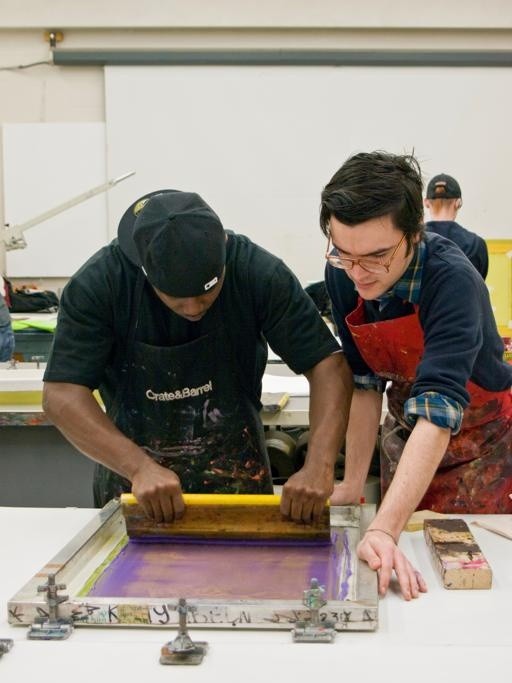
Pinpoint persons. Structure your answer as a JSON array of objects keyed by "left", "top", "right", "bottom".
[
  {"left": 40, "top": 186, "right": 357, "bottom": 525},
  {"left": 314, "top": 147, "right": 511, "bottom": 600},
  {"left": 419, "top": 171, "right": 489, "bottom": 282}
]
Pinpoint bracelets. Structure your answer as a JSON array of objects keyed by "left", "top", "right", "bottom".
[{"left": 365, "top": 526, "right": 399, "bottom": 546}]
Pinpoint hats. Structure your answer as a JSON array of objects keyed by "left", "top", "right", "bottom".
[
  {"left": 428, "top": 174, "right": 461, "bottom": 198},
  {"left": 118, "top": 189, "right": 226, "bottom": 296}
]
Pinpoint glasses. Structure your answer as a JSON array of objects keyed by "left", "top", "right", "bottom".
[{"left": 325, "top": 235, "right": 406, "bottom": 273}]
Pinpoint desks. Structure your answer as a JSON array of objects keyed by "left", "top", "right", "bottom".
[{"left": 0, "top": 310, "right": 512, "bottom": 683}]
